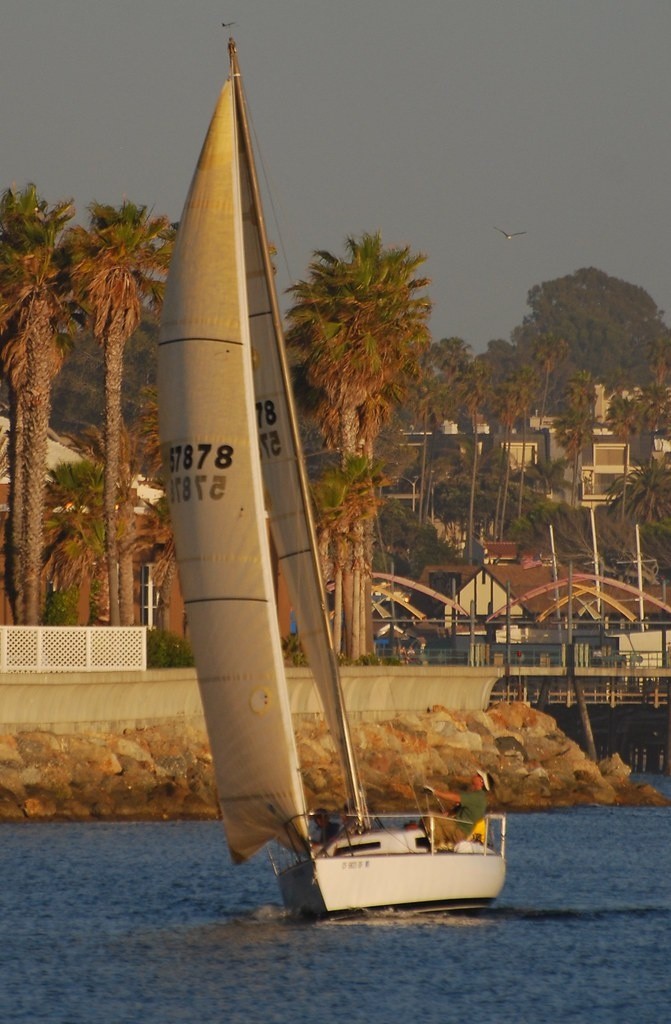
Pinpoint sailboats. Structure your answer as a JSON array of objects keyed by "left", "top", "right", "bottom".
[{"left": 157, "top": 36, "right": 509, "bottom": 917}]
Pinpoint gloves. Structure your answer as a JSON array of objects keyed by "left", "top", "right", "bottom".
[{"left": 421, "top": 786, "right": 435, "bottom": 796}]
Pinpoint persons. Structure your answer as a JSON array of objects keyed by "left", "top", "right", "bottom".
[
  {"left": 421, "top": 768, "right": 495, "bottom": 844},
  {"left": 314, "top": 808, "right": 339, "bottom": 842}
]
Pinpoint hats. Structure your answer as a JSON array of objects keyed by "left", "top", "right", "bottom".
[{"left": 478, "top": 768, "right": 494, "bottom": 793}]
